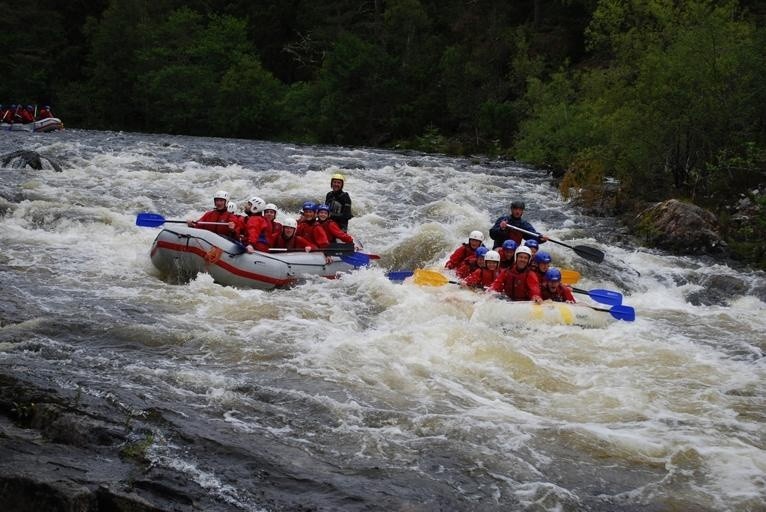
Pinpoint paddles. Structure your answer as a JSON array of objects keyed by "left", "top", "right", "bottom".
[
  {"left": 135, "top": 213, "right": 229, "bottom": 227},
  {"left": 503, "top": 218, "right": 604, "bottom": 264},
  {"left": 339, "top": 251, "right": 370, "bottom": 267},
  {"left": 382, "top": 267, "right": 450, "bottom": 279},
  {"left": 267, "top": 242, "right": 355, "bottom": 255},
  {"left": 558, "top": 271, "right": 580, "bottom": 286},
  {"left": 589, "top": 305, "right": 635, "bottom": 320},
  {"left": 364, "top": 253, "right": 381, "bottom": 260},
  {"left": 8, "top": 107, "right": 20, "bottom": 133},
  {"left": 570, "top": 286, "right": 622, "bottom": 307},
  {"left": 32, "top": 105, "right": 37, "bottom": 132},
  {"left": 414, "top": 268, "right": 486, "bottom": 292}
]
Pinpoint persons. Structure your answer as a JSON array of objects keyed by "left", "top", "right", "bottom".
[
  {"left": 186, "top": 189, "right": 354, "bottom": 266},
  {"left": 325, "top": 173, "right": 354, "bottom": 233},
  {"left": 1, "top": 105, "right": 54, "bottom": 125},
  {"left": 489, "top": 199, "right": 549, "bottom": 250},
  {"left": 444, "top": 230, "right": 576, "bottom": 304}
]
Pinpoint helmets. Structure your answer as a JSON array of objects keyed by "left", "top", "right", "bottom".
[
  {"left": 332, "top": 175, "right": 345, "bottom": 180},
  {"left": 213, "top": 190, "right": 331, "bottom": 215},
  {"left": 469, "top": 231, "right": 562, "bottom": 279},
  {"left": 510, "top": 201, "right": 524, "bottom": 207}
]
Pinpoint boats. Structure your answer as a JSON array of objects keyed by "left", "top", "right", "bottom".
[
  {"left": 404, "top": 276, "right": 611, "bottom": 331},
  {"left": 150, "top": 225, "right": 368, "bottom": 292},
  {"left": 0, "top": 118, "right": 65, "bottom": 132}
]
